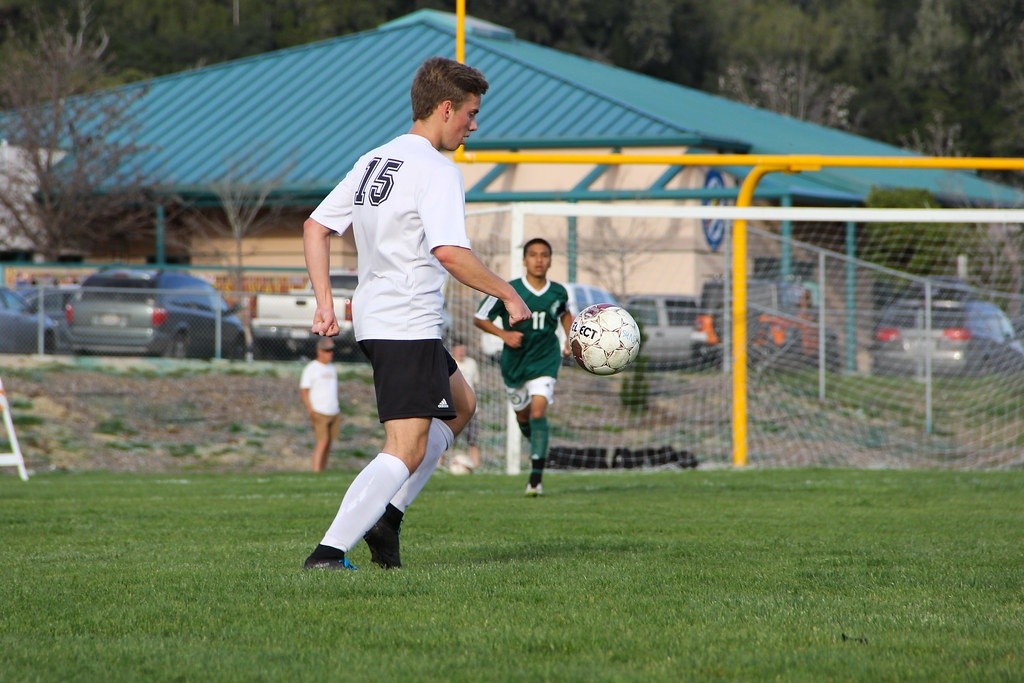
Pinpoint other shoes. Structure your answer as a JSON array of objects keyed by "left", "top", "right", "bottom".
[
  {"left": 525, "top": 473, "right": 543, "bottom": 497},
  {"left": 544, "top": 436, "right": 552, "bottom": 469}
]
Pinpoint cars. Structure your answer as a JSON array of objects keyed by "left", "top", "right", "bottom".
[{"left": 870, "top": 277, "right": 1024, "bottom": 379}]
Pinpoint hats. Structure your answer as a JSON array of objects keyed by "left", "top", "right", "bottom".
[
  {"left": 452, "top": 333, "right": 468, "bottom": 345},
  {"left": 317, "top": 336, "right": 336, "bottom": 350}
]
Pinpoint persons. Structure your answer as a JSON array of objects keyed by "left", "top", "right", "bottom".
[
  {"left": 473, "top": 238, "right": 573, "bottom": 495},
  {"left": 300, "top": 336, "right": 340, "bottom": 472},
  {"left": 441, "top": 336, "right": 481, "bottom": 474},
  {"left": 303, "top": 57, "right": 533, "bottom": 569}
]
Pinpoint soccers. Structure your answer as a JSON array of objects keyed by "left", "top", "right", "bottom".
[{"left": 571, "top": 305, "right": 642, "bottom": 373}]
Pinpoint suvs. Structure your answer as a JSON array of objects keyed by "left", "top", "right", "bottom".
[
  {"left": 480, "top": 279, "right": 838, "bottom": 373},
  {"left": 0, "top": 264, "right": 248, "bottom": 361}
]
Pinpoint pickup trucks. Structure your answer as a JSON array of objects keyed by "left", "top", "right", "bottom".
[{"left": 248, "top": 269, "right": 452, "bottom": 362}]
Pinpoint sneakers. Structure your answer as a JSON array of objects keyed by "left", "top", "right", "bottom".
[
  {"left": 304, "top": 557, "right": 360, "bottom": 574},
  {"left": 363, "top": 517, "right": 404, "bottom": 570}
]
